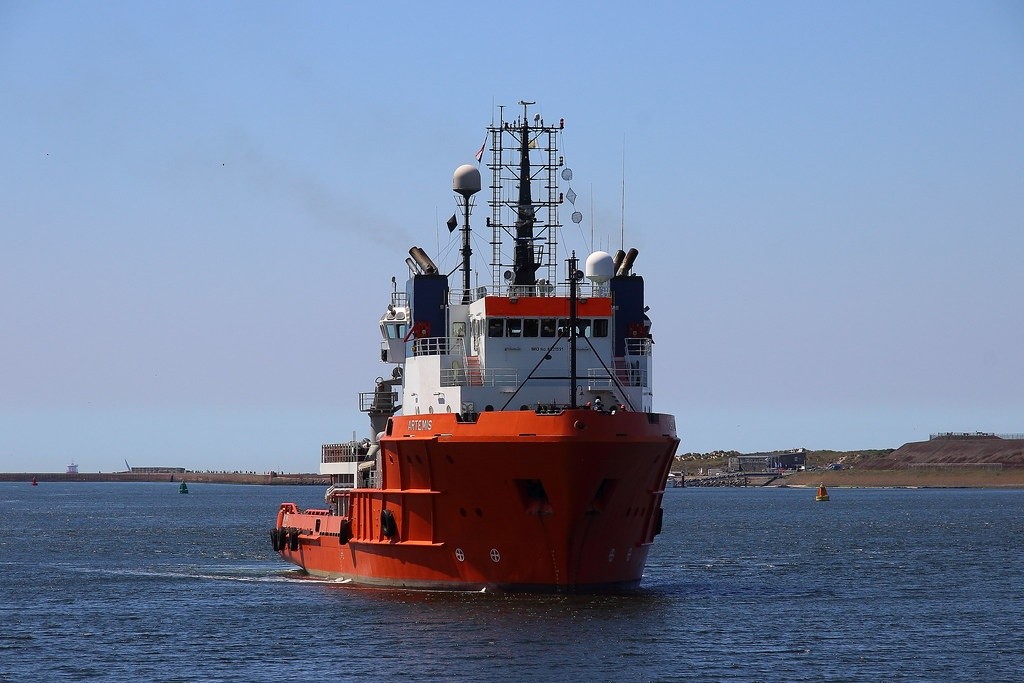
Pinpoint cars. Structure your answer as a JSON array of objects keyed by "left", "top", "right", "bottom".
[{"left": 827, "top": 464, "right": 842, "bottom": 470}]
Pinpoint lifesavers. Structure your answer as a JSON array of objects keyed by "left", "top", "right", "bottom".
[
  {"left": 269, "top": 527, "right": 279, "bottom": 553},
  {"left": 276, "top": 526, "right": 287, "bottom": 550},
  {"left": 288, "top": 527, "right": 299, "bottom": 551},
  {"left": 653, "top": 507, "right": 664, "bottom": 534},
  {"left": 380, "top": 508, "right": 396, "bottom": 537},
  {"left": 338, "top": 518, "right": 349, "bottom": 544}
]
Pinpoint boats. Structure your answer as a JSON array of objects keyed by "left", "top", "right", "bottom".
[{"left": 266, "top": 100, "right": 681, "bottom": 594}]
